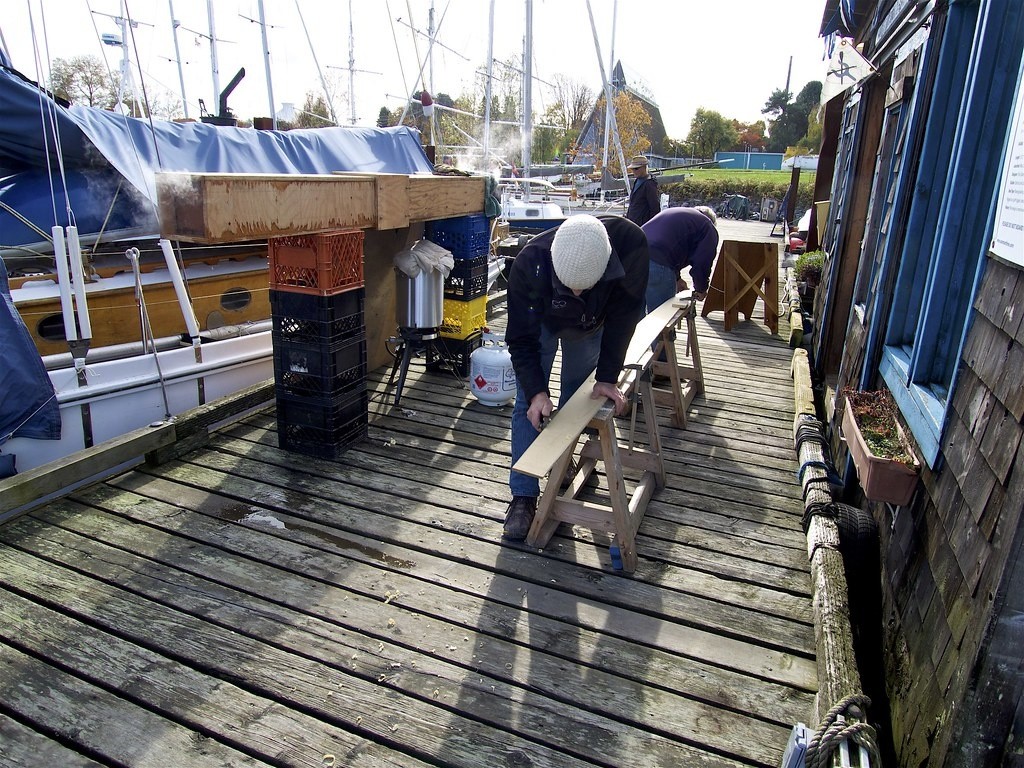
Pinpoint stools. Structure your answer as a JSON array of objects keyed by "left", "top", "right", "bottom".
[
  {"left": 612, "top": 297, "right": 705, "bottom": 430},
  {"left": 521, "top": 349, "right": 668, "bottom": 575}
]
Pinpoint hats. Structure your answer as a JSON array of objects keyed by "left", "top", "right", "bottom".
[
  {"left": 628, "top": 156, "right": 649, "bottom": 169},
  {"left": 551, "top": 214, "right": 612, "bottom": 290}
]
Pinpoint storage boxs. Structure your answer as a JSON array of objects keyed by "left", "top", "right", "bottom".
[
  {"left": 276, "top": 386, "right": 369, "bottom": 460},
  {"left": 444, "top": 255, "right": 490, "bottom": 304},
  {"left": 268, "top": 286, "right": 368, "bottom": 347},
  {"left": 266, "top": 227, "right": 367, "bottom": 297},
  {"left": 270, "top": 329, "right": 369, "bottom": 406},
  {"left": 422, "top": 213, "right": 492, "bottom": 260},
  {"left": 425, "top": 328, "right": 485, "bottom": 380},
  {"left": 438, "top": 289, "right": 488, "bottom": 340}
]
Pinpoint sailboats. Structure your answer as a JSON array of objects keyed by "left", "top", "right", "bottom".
[{"left": 0, "top": 0, "right": 668, "bottom": 525}]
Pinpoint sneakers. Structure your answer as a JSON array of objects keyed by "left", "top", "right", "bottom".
[
  {"left": 547, "top": 456, "right": 578, "bottom": 487},
  {"left": 503, "top": 496, "right": 537, "bottom": 538}
]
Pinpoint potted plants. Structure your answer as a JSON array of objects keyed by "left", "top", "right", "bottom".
[{"left": 793, "top": 248, "right": 825, "bottom": 317}]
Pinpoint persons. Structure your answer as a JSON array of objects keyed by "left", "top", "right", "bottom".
[
  {"left": 639, "top": 206, "right": 719, "bottom": 373},
  {"left": 503, "top": 216, "right": 649, "bottom": 543},
  {"left": 625, "top": 155, "right": 661, "bottom": 227}
]
